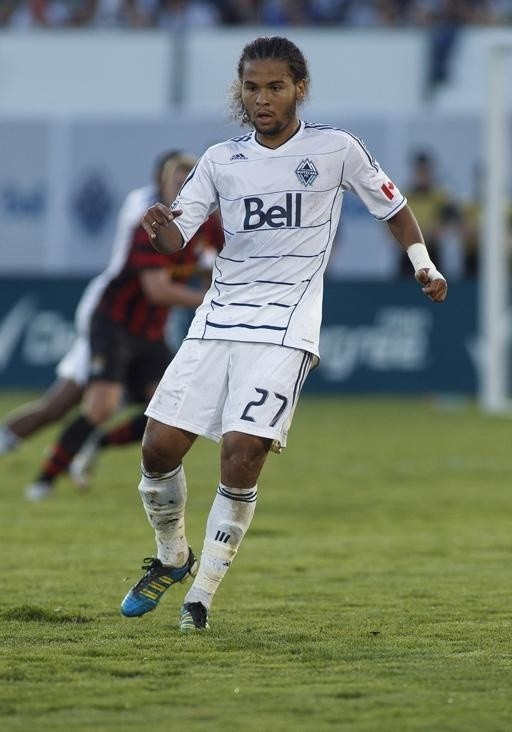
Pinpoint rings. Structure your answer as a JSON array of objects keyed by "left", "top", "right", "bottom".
[{"left": 151, "top": 221, "right": 157, "bottom": 226}]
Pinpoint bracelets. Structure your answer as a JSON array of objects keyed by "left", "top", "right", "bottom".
[{"left": 405, "top": 243, "right": 437, "bottom": 271}]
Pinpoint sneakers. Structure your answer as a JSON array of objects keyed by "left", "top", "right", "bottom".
[
  {"left": 179, "top": 601, "right": 210, "bottom": 636},
  {"left": 24, "top": 441, "right": 99, "bottom": 503},
  {"left": 120, "top": 545, "right": 198, "bottom": 618}
]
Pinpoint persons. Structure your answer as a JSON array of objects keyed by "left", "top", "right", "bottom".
[
  {"left": 1, "top": 1, "right": 511, "bottom": 30},
  {"left": 397, "top": 152, "right": 452, "bottom": 284},
  {"left": 118, "top": 35, "right": 450, "bottom": 633},
  {"left": 459, "top": 157, "right": 478, "bottom": 275},
  {"left": 0, "top": 150, "right": 184, "bottom": 449},
  {"left": 21, "top": 159, "right": 225, "bottom": 503}
]
